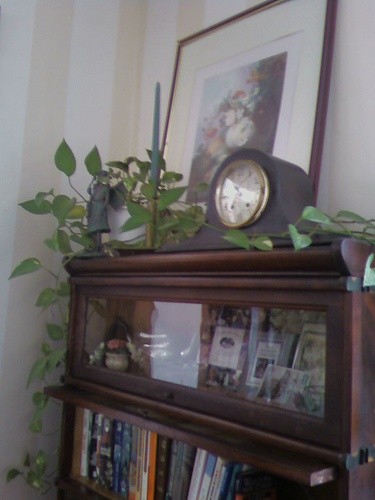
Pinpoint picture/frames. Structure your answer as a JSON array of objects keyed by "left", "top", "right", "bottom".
[{"left": 156, "top": 0, "right": 336, "bottom": 249}]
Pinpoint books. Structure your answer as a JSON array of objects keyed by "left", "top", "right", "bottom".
[{"left": 69, "top": 406, "right": 248, "bottom": 500}]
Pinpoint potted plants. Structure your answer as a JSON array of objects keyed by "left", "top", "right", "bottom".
[{"left": 5, "top": 137, "right": 374, "bottom": 492}]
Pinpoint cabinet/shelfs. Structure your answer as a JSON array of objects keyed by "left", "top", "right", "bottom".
[{"left": 42, "top": 237, "right": 374, "bottom": 500}]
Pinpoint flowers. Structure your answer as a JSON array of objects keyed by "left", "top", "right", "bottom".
[{"left": 107, "top": 338, "right": 127, "bottom": 352}]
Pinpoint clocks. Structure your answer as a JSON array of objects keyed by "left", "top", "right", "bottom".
[{"left": 192, "top": 149, "right": 308, "bottom": 244}]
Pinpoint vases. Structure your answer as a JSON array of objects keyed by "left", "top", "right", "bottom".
[{"left": 106, "top": 354, "right": 129, "bottom": 372}]
